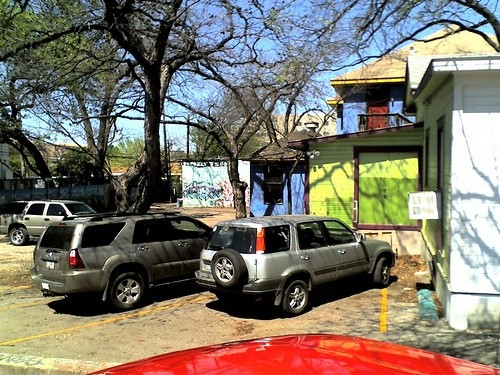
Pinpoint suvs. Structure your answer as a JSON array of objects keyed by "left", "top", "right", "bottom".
[
  {"left": 28, "top": 209, "right": 215, "bottom": 311},
  {"left": 196, "top": 211, "right": 396, "bottom": 312},
  {"left": 1, "top": 200, "right": 95, "bottom": 244}
]
